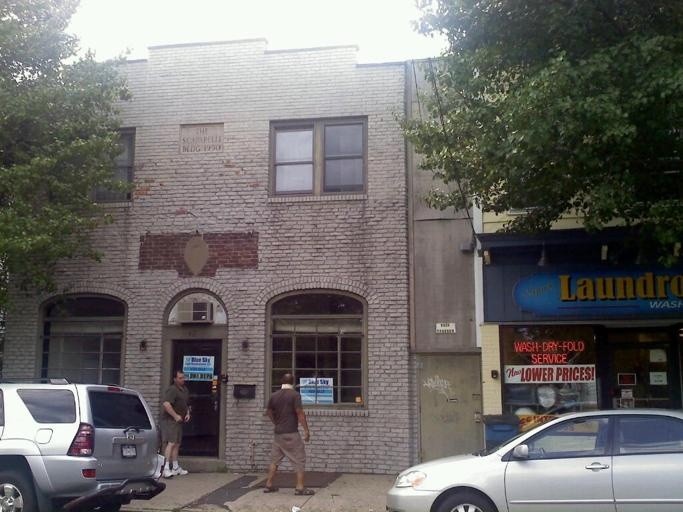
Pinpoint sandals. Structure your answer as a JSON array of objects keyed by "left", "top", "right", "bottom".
[
  {"left": 263, "top": 486, "right": 279, "bottom": 493},
  {"left": 295, "top": 487, "right": 314, "bottom": 496}
]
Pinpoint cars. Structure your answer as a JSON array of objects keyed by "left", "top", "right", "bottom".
[
  {"left": 386, "top": 409, "right": 683, "bottom": 512},
  {"left": 0, "top": 383, "right": 166, "bottom": 512}
]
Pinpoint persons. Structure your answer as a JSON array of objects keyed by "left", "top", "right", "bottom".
[
  {"left": 263, "top": 373, "right": 315, "bottom": 494},
  {"left": 160, "top": 371, "right": 192, "bottom": 477}
]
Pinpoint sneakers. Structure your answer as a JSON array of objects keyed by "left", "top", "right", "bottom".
[
  {"left": 162, "top": 470, "right": 172, "bottom": 478},
  {"left": 172, "top": 466, "right": 188, "bottom": 475}
]
